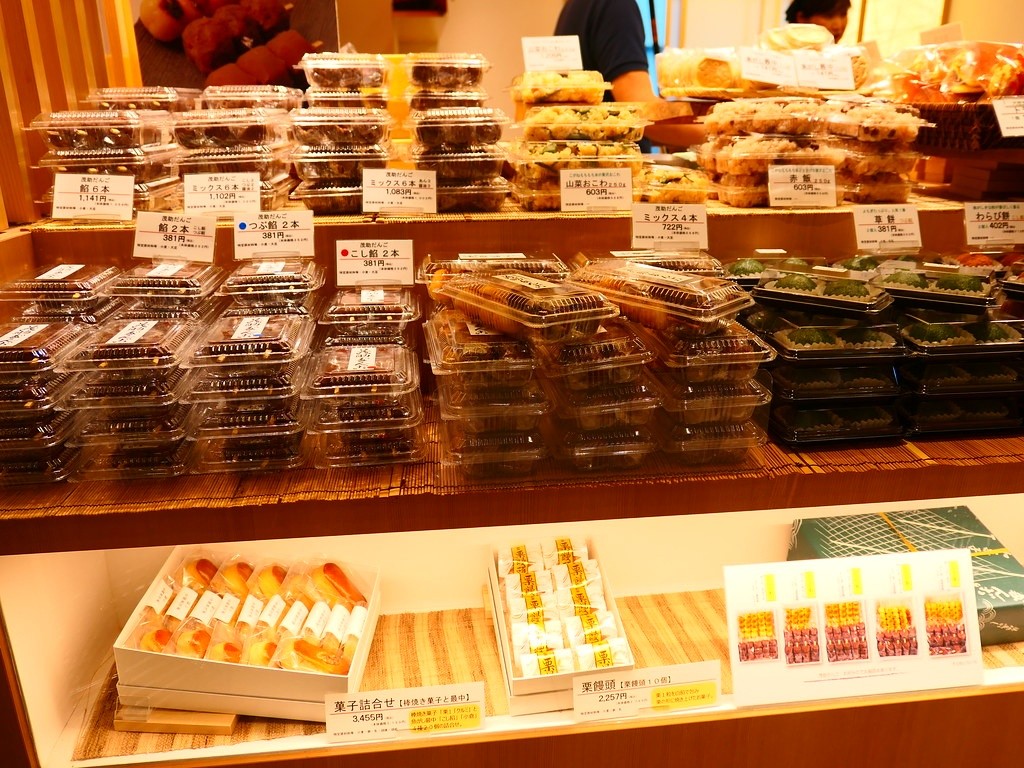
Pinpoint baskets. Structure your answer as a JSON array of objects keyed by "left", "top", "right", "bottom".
[{"left": 911, "top": 101, "right": 1024, "bottom": 152}]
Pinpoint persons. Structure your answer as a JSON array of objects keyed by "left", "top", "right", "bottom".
[
  {"left": 556, "top": 0, "right": 706, "bottom": 152},
  {"left": 786, "top": 0, "right": 851, "bottom": 44}
]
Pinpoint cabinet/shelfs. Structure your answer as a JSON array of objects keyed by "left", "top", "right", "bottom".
[{"left": 0, "top": 189, "right": 1024, "bottom": 768}]
